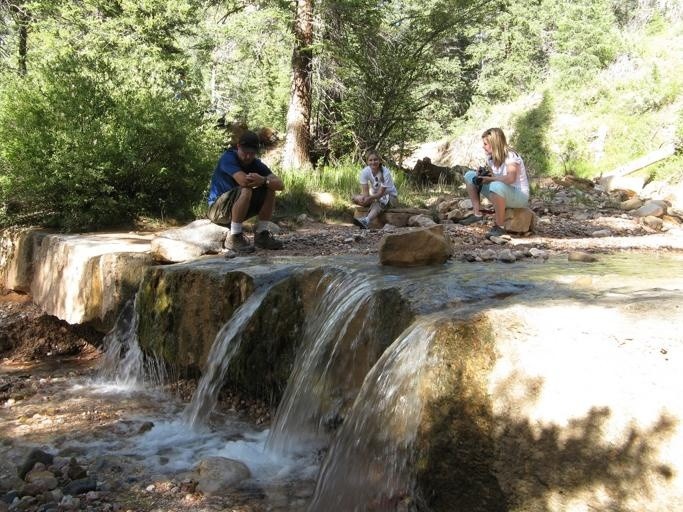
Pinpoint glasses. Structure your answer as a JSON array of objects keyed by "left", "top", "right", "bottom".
[{"left": 374, "top": 177, "right": 378, "bottom": 188}]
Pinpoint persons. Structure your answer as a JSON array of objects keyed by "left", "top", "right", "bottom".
[
  {"left": 204, "top": 130, "right": 286, "bottom": 252},
  {"left": 345, "top": 149, "right": 398, "bottom": 228},
  {"left": 453, "top": 127, "right": 528, "bottom": 241}
]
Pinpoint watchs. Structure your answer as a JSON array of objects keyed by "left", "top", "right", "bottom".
[{"left": 264, "top": 177, "right": 268, "bottom": 186}]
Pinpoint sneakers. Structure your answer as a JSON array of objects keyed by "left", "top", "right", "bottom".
[
  {"left": 254, "top": 230, "right": 282, "bottom": 249},
  {"left": 352, "top": 217, "right": 369, "bottom": 230},
  {"left": 224, "top": 232, "right": 255, "bottom": 253},
  {"left": 485, "top": 225, "right": 506, "bottom": 240},
  {"left": 459, "top": 214, "right": 483, "bottom": 226}
]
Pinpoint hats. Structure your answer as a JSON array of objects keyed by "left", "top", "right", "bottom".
[{"left": 239, "top": 131, "right": 259, "bottom": 153}]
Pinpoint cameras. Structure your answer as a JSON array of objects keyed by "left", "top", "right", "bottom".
[{"left": 473, "top": 166, "right": 491, "bottom": 186}]
